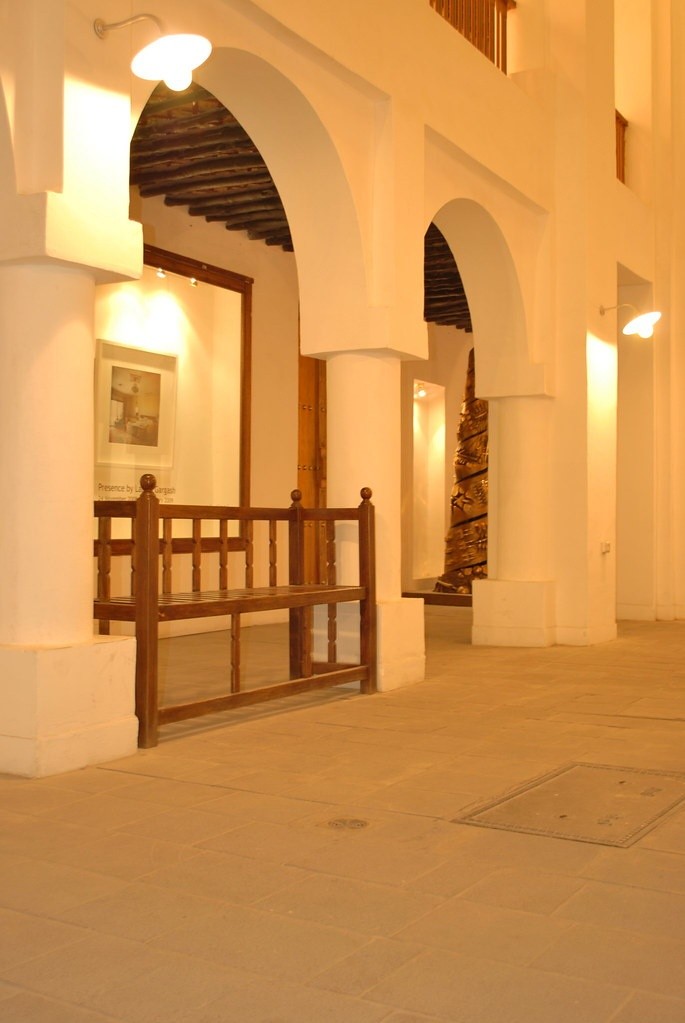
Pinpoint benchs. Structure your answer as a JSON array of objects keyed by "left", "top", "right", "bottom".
[{"left": 94, "top": 477, "right": 380, "bottom": 747}]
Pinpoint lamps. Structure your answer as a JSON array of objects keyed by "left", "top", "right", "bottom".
[
  {"left": 600, "top": 303, "right": 662, "bottom": 338},
  {"left": 94, "top": 14, "right": 213, "bottom": 91}
]
